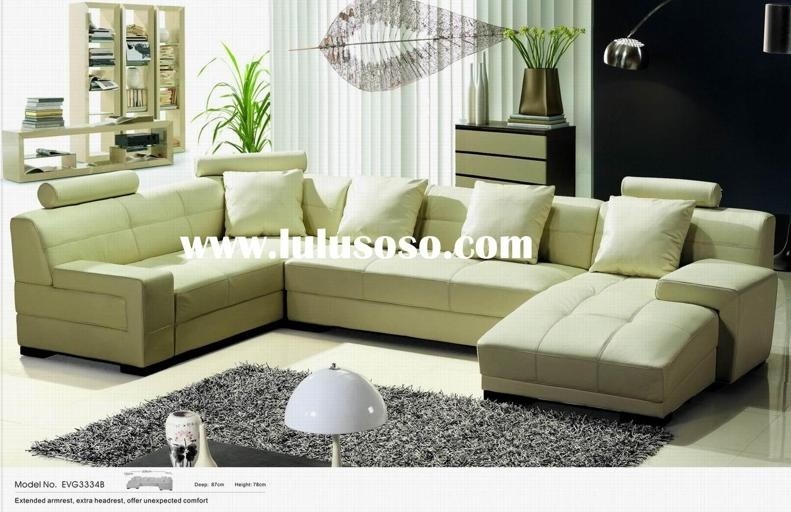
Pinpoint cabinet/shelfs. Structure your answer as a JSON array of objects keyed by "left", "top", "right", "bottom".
[
  {"left": 2, "top": 0, "right": 185, "bottom": 184},
  {"left": 455, "top": 120, "right": 576, "bottom": 197}
]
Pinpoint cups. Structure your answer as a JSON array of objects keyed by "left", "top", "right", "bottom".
[
  {"left": 164, "top": 411, "right": 201, "bottom": 467},
  {"left": 110, "top": 146, "right": 127, "bottom": 163}
]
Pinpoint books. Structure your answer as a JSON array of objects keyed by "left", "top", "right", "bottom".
[
  {"left": 89, "top": 25, "right": 115, "bottom": 67},
  {"left": 160, "top": 37, "right": 178, "bottom": 106},
  {"left": 127, "top": 87, "right": 147, "bottom": 108},
  {"left": 126, "top": 36, "right": 151, "bottom": 65},
  {"left": 21, "top": 97, "right": 65, "bottom": 131},
  {"left": 109, "top": 116, "right": 153, "bottom": 123},
  {"left": 507, "top": 114, "right": 570, "bottom": 130}
]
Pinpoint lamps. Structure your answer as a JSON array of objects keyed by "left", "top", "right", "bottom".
[
  {"left": 760, "top": 3, "right": 790, "bottom": 53},
  {"left": 603, "top": 1, "right": 669, "bottom": 71},
  {"left": 285, "top": 363, "right": 387, "bottom": 467}
]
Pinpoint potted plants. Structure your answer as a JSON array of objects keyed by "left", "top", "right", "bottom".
[{"left": 503, "top": 23, "right": 586, "bottom": 118}]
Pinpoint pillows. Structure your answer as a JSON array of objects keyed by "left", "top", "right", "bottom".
[
  {"left": 585, "top": 195, "right": 695, "bottom": 278},
  {"left": 336, "top": 175, "right": 429, "bottom": 248},
  {"left": 221, "top": 168, "right": 308, "bottom": 239},
  {"left": 454, "top": 177, "right": 556, "bottom": 265}
]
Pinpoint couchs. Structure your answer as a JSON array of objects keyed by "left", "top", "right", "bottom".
[{"left": 10, "top": 149, "right": 777, "bottom": 421}]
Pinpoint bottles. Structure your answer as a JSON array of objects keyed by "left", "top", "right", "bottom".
[
  {"left": 126, "top": 67, "right": 141, "bottom": 88},
  {"left": 465, "top": 50, "right": 490, "bottom": 126},
  {"left": 192, "top": 424, "right": 218, "bottom": 467}
]
